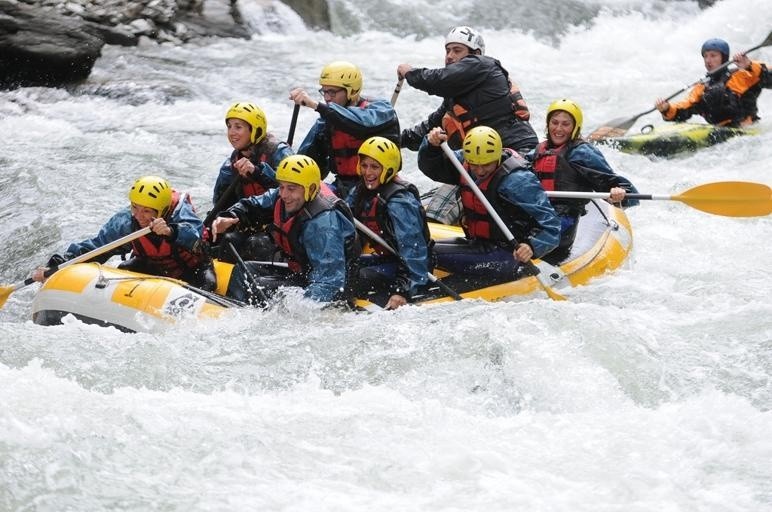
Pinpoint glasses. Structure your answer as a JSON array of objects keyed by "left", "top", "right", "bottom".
[{"left": 318, "top": 87, "right": 343, "bottom": 96}]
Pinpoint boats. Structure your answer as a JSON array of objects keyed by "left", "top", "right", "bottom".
[
  {"left": 594, "top": 110, "right": 772, "bottom": 161},
  {"left": 31, "top": 193, "right": 636, "bottom": 336}
]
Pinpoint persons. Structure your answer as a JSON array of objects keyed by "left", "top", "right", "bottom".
[
  {"left": 418, "top": 125, "right": 562, "bottom": 285},
  {"left": 204, "top": 103, "right": 297, "bottom": 266},
  {"left": 32, "top": 176, "right": 217, "bottom": 294},
  {"left": 210, "top": 154, "right": 362, "bottom": 312},
  {"left": 343, "top": 136, "right": 438, "bottom": 310},
  {"left": 655, "top": 39, "right": 772, "bottom": 128},
  {"left": 524, "top": 98, "right": 640, "bottom": 266},
  {"left": 289, "top": 62, "right": 402, "bottom": 200},
  {"left": 399, "top": 26, "right": 539, "bottom": 225}
]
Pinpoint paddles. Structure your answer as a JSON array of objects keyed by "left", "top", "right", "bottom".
[
  {"left": 1, "top": 226, "right": 153, "bottom": 308},
  {"left": 441, "top": 132, "right": 573, "bottom": 300},
  {"left": 586, "top": 30, "right": 772, "bottom": 141},
  {"left": 545, "top": 181, "right": 771, "bottom": 217}
]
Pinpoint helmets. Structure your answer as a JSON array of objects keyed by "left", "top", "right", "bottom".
[
  {"left": 546, "top": 98, "right": 583, "bottom": 141},
  {"left": 701, "top": 39, "right": 729, "bottom": 64},
  {"left": 445, "top": 26, "right": 485, "bottom": 55},
  {"left": 356, "top": 136, "right": 401, "bottom": 185},
  {"left": 463, "top": 125, "right": 502, "bottom": 167},
  {"left": 226, "top": 102, "right": 266, "bottom": 145},
  {"left": 129, "top": 176, "right": 173, "bottom": 218},
  {"left": 276, "top": 154, "right": 321, "bottom": 202},
  {"left": 319, "top": 62, "right": 362, "bottom": 101}
]
